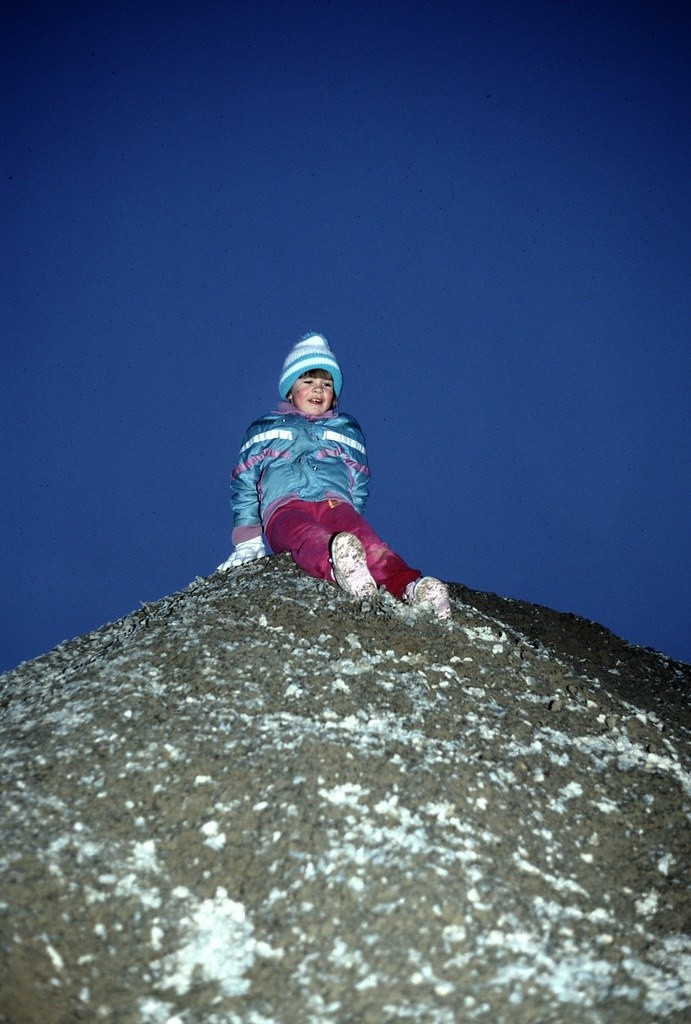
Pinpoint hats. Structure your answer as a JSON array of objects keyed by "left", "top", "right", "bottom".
[{"left": 279, "top": 331, "right": 342, "bottom": 401}]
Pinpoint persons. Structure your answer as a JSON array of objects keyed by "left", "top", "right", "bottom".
[{"left": 217, "top": 332, "right": 451, "bottom": 620}]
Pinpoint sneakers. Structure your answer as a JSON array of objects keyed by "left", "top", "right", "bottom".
[
  {"left": 404, "top": 576, "right": 452, "bottom": 621},
  {"left": 329, "top": 531, "right": 377, "bottom": 598},
  {"left": 217, "top": 535, "right": 266, "bottom": 571}
]
